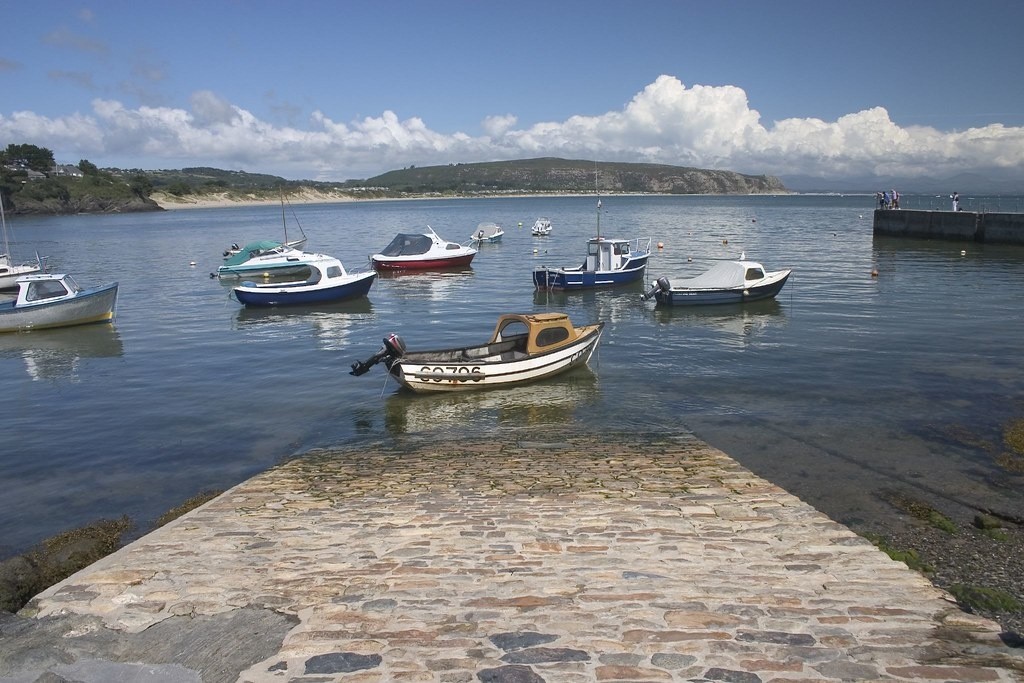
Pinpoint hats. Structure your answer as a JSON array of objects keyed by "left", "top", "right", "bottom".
[{"left": 953, "top": 192, "right": 957, "bottom": 194}]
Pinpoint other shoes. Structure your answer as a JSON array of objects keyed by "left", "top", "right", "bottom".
[
  {"left": 891, "top": 208, "right": 895, "bottom": 210},
  {"left": 896, "top": 208, "right": 900, "bottom": 210}
]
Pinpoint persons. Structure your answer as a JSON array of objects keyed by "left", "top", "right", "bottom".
[
  {"left": 877, "top": 189, "right": 901, "bottom": 210},
  {"left": 952, "top": 191, "right": 959, "bottom": 212}
]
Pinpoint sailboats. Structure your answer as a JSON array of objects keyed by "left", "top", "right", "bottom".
[{"left": 0, "top": 192, "right": 41, "bottom": 293}]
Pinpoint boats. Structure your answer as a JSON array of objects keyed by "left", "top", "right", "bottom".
[
  {"left": 209, "top": 183, "right": 378, "bottom": 306},
  {"left": 348, "top": 312, "right": 606, "bottom": 394},
  {"left": 371, "top": 224, "right": 481, "bottom": 271},
  {"left": 640, "top": 249, "right": 792, "bottom": 307},
  {"left": 531, "top": 216, "right": 552, "bottom": 236},
  {"left": 533, "top": 196, "right": 652, "bottom": 291},
  {"left": 470, "top": 222, "right": 504, "bottom": 244},
  {"left": 0, "top": 275, "right": 118, "bottom": 334}
]
